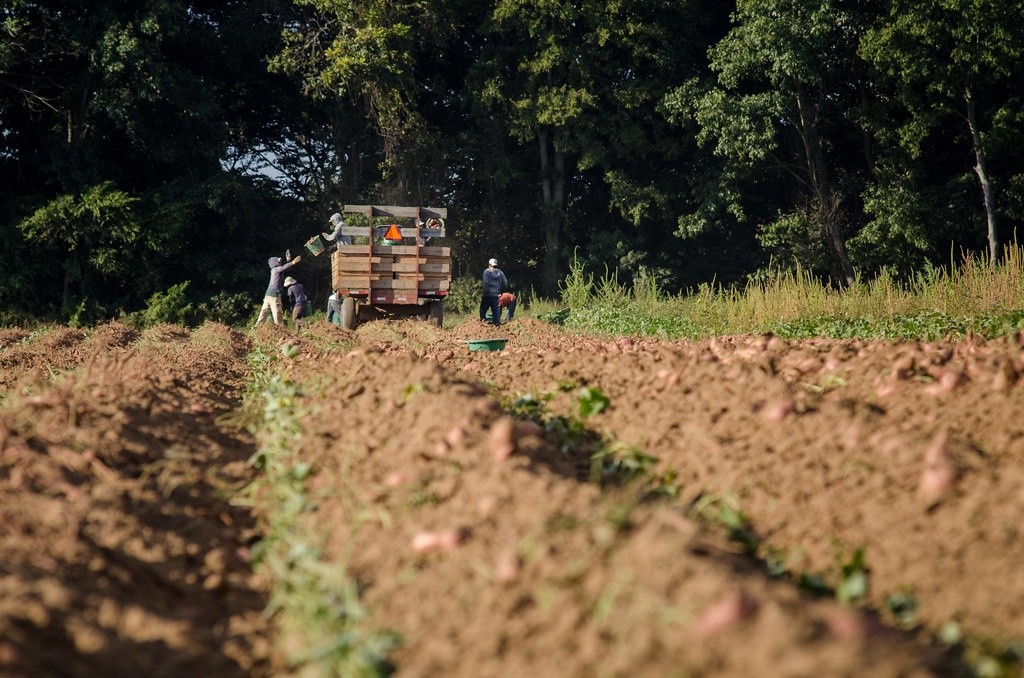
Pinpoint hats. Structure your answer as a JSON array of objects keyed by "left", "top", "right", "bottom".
[
  {"left": 489, "top": 259, "right": 498, "bottom": 266},
  {"left": 284, "top": 276, "right": 298, "bottom": 286},
  {"left": 328, "top": 212, "right": 342, "bottom": 222},
  {"left": 268, "top": 257, "right": 280, "bottom": 268}
]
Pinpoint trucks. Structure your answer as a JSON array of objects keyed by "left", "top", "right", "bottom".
[{"left": 330, "top": 205, "right": 451, "bottom": 329}]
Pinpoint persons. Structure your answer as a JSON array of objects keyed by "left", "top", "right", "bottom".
[
  {"left": 496, "top": 292, "right": 517, "bottom": 320},
  {"left": 479, "top": 258, "right": 508, "bottom": 325},
  {"left": 416, "top": 220, "right": 432, "bottom": 247},
  {"left": 284, "top": 276, "right": 311, "bottom": 319},
  {"left": 253, "top": 256, "right": 302, "bottom": 327},
  {"left": 322, "top": 213, "right": 353, "bottom": 250}
]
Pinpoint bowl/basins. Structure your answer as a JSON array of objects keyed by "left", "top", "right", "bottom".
[{"left": 466, "top": 339, "right": 507, "bottom": 352}]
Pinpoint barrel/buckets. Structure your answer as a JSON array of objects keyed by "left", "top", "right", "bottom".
[
  {"left": 303, "top": 303, "right": 312, "bottom": 317},
  {"left": 332, "top": 312, "right": 341, "bottom": 326},
  {"left": 303, "top": 235, "right": 325, "bottom": 256},
  {"left": 426, "top": 218, "right": 443, "bottom": 230}
]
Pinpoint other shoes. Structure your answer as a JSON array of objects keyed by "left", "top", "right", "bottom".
[{"left": 493, "top": 322, "right": 501, "bottom": 326}]
